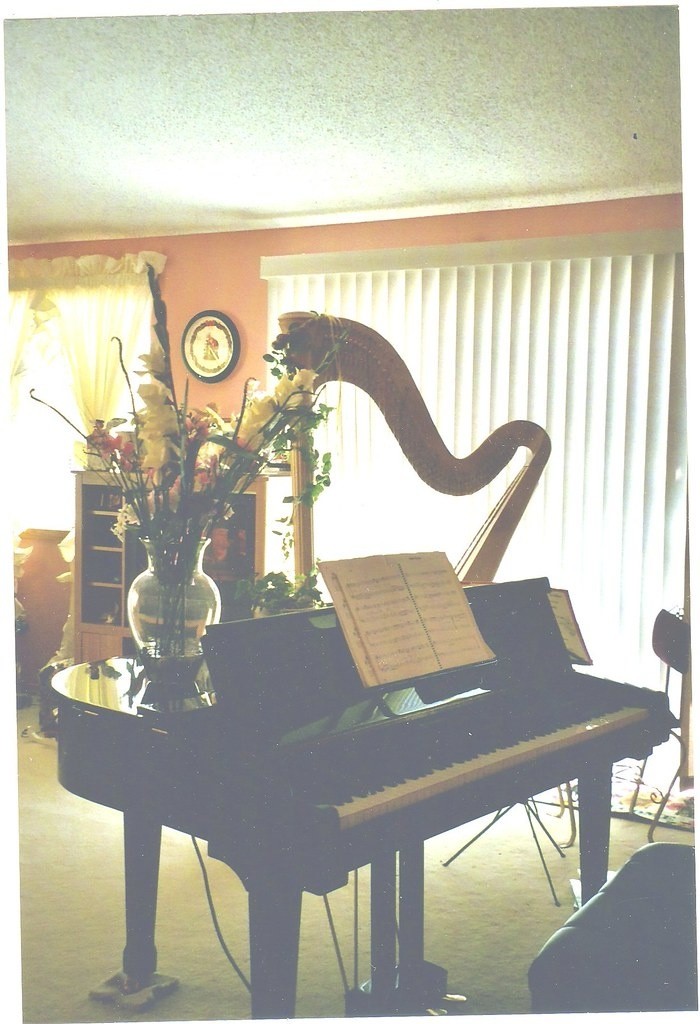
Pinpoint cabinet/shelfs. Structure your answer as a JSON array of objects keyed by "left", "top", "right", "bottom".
[{"left": 72, "top": 472, "right": 269, "bottom": 664}]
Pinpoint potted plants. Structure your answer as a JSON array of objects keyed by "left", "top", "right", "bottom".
[{"left": 234, "top": 558, "right": 327, "bottom": 618}]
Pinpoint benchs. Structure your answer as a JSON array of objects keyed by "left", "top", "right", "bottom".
[{"left": 527, "top": 842, "right": 698, "bottom": 1011}]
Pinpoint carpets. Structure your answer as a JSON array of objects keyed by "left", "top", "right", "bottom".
[{"left": 529, "top": 780, "right": 695, "bottom": 833}]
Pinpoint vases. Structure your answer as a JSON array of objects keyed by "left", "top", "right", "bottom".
[
  {"left": 181, "top": 310, "right": 240, "bottom": 384},
  {"left": 127, "top": 535, "right": 223, "bottom": 681}
]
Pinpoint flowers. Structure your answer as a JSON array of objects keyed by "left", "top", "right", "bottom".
[{"left": 29, "top": 262, "right": 337, "bottom": 660}]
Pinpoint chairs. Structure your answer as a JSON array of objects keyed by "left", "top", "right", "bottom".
[{"left": 555, "top": 610, "right": 691, "bottom": 848}]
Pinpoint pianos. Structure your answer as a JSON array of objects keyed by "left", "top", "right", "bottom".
[{"left": 45, "top": 576, "right": 680, "bottom": 1019}]
